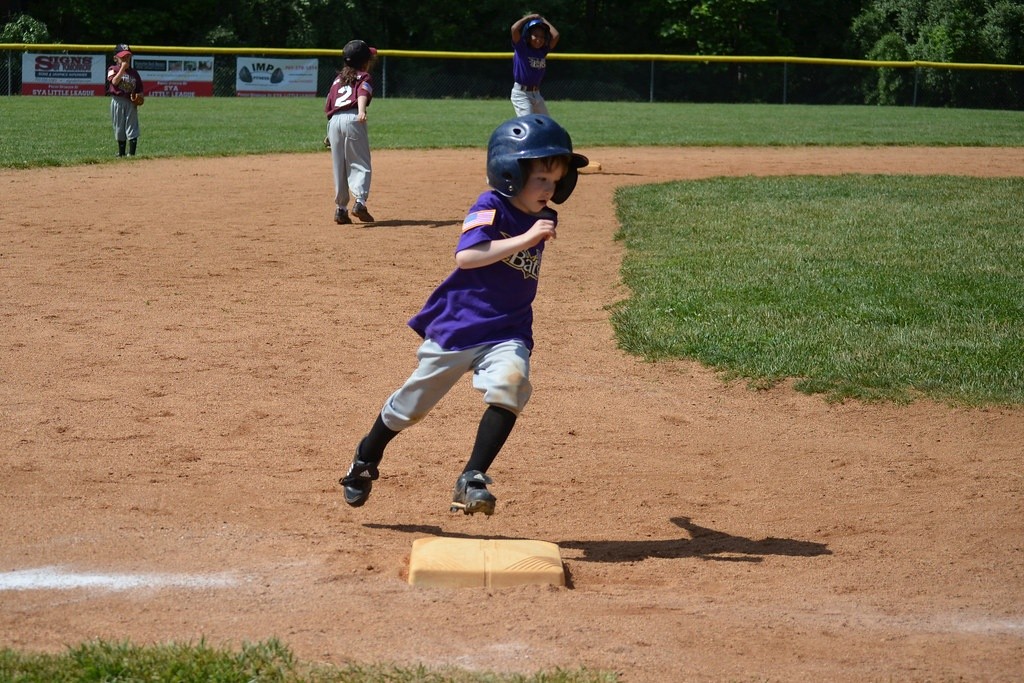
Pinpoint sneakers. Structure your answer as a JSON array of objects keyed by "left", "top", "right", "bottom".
[
  {"left": 449, "top": 470, "right": 497, "bottom": 520},
  {"left": 338, "top": 438, "right": 379, "bottom": 508}
]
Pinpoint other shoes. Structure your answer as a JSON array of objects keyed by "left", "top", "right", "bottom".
[
  {"left": 334, "top": 209, "right": 352, "bottom": 224},
  {"left": 351, "top": 202, "right": 374, "bottom": 223}
]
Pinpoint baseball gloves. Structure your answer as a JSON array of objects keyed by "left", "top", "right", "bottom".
[{"left": 130, "top": 93, "right": 144, "bottom": 105}]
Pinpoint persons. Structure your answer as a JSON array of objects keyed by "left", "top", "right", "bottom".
[
  {"left": 107, "top": 45, "right": 144, "bottom": 156},
  {"left": 339, "top": 113, "right": 589, "bottom": 516},
  {"left": 511, "top": 14, "right": 559, "bottom": 117},
  {"left": 323, "top": 40, "right": 377, "bottom": 224}
]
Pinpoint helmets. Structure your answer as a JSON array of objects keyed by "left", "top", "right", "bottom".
[
  {"left": 522, "top": 19, "right": 550, "bottom": 42},
  {"left": 486, "top": 114, "right": 589, "bottom": 204}
]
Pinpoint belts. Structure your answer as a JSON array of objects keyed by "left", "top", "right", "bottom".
[{"left": 521, "top": 85, "right": 538, "bottom": 91}]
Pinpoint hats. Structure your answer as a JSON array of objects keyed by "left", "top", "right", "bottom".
[
  {"left": 343, "top": 40, "right": 377, "bottom": 65},
  {"left": 114, "top": 44, "right": 132, "bottom": 58}
]
